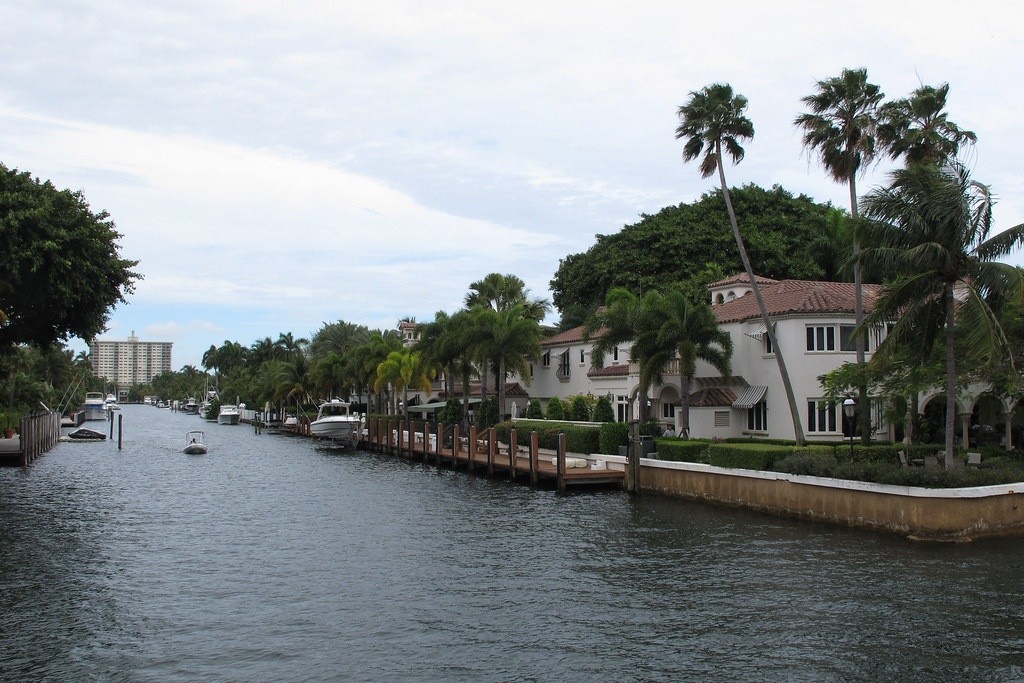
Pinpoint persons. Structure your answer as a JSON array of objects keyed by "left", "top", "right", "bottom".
[{"left": 663, "top": 424, "right": 674, "bottom": 437}]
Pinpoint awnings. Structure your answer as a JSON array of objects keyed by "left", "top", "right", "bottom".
[
  {"left": 732, "top": 385, "right": 768, "bottom": 410},
  {"left": 407, "top": 398, "right": 491, "bottom": 412},
  {"left": 748, "top": 321, "right": 778, "bottom": 338}
]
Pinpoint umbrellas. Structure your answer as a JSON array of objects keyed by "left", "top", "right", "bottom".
[{"left": 510, "top": 402, "right": 517, "bottom": 422}]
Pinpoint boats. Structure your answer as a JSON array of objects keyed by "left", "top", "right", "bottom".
[
  {"left": 217, "top": 403, "right": 241, "bottom": 426},
  {"left": 68, "top": 426, "right": 107, "bottom": 440},
  {"left": 156, "top": 376, "right": 219, "bottom": 418},
  {"left": 104, "top": 392, "right": 122, "bottom": 411},
  {"left": 183, "top": 430, "right": 209, "bottom": 454},
  {"left": 310, "top": 396, "right": 362, "bottom": 444},
  {"left": 82, "top": 391, "right": 109, "bottom": 421},
  {"left": 284, "top": 416, "right": 298, "bottom": 429}
]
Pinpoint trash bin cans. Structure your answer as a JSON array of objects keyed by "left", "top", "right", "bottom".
[{"left": 637, "top": 434, "right": 657, "bottom": 459}]
